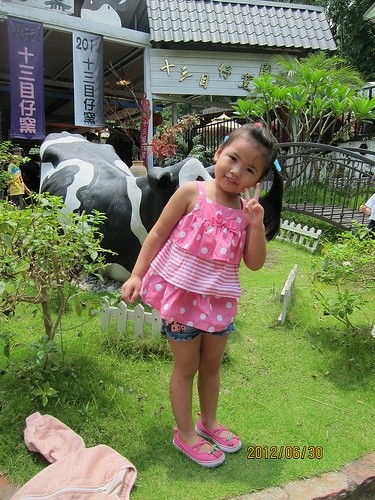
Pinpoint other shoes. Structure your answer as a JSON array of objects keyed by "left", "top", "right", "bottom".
[
  {"left": 171, "top": 428, "right": 225, "bottom": 467},
  {"left": 195, "top": 419, "right": 242, "bottom": 453}
]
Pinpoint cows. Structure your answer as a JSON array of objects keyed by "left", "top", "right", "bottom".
[{"left": 39, "top": 130, "right": 215, "bottom": 284}]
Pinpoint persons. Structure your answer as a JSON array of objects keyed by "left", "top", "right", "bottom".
[
  {"left": 360, "top": 193, "right": 375, "bottom": 239},
  {"left": 88, "top": 133, "right": 99, "bottom": 144},
  {"left": 7, "top": 146, "right": 31, "bottom": 209},
  {"left": 121, "top": 123, "right": 284, "bottom": 468}
]
